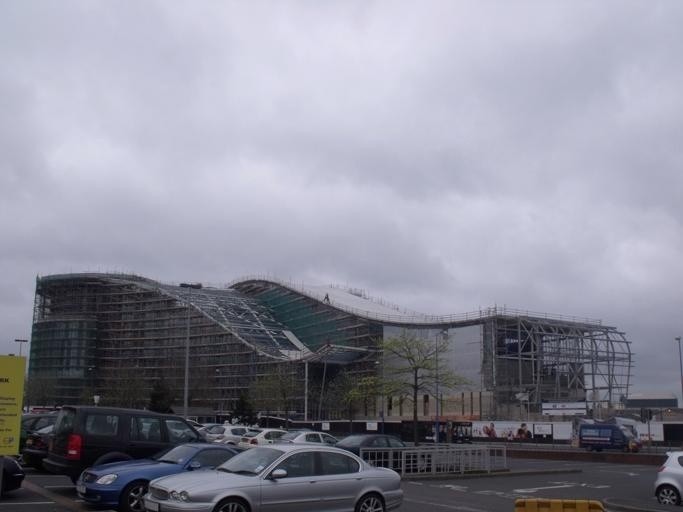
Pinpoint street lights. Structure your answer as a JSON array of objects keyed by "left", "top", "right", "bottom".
[
  {"left": 14, "top": 339, "right": 27, "bottom": 357},
  {"left": 435, "top": 328, "right": 449, "bottom": 444},
  {"left": 674, "top": 337, "right": 683, "bottom": 401},
  {"left": 179, "top": 282, "right": 203, "bottom": 417}
]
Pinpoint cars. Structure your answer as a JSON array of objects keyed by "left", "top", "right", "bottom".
[{"left": 652, "top": 450, "right": 682, "bottom": 507}]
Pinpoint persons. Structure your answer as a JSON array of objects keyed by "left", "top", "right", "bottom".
[
  {"left": 507, "top": 430, "right": 513, "bottom": 447},
  {"left": 514, "top": 422, "right": 532, "bottom": 438},
  {"left": 486, "top": 422, "right": 497, "bottom": 438}
]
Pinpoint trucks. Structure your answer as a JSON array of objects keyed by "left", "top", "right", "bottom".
[{"left": 578, "top": 423, "right": 642, "bottom": 454}]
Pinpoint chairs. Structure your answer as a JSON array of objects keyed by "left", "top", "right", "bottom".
[{"left": 138, "top": 422, "right": 160, "bottom": 441}]
